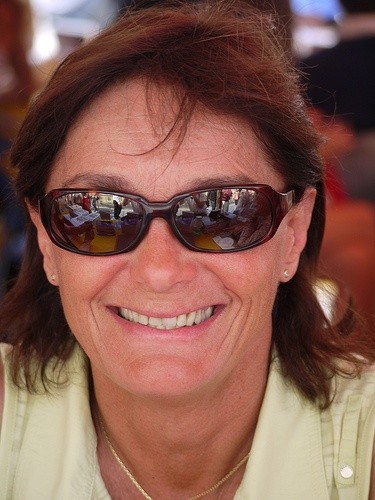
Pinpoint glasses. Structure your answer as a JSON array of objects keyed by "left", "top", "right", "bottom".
[{"left": 29, "top": 184, "right": 296, "bottom": 256}]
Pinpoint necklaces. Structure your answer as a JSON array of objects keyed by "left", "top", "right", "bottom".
[{"left": 98, "top": 410, "right": 251, "bottom": 500}]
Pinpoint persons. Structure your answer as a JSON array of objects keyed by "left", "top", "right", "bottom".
[{"left": 1, "top": 5, "right": 375, "bottom": 500}]
